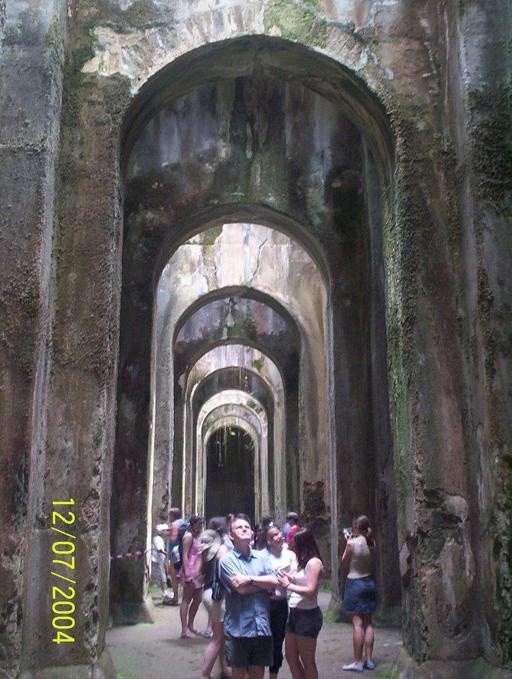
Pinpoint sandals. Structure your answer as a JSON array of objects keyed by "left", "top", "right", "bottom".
[
  {"left": 342, "top": 661, "right": 374, "bottom": 672},
  {"left": 181, "top": 630, "right": 203, "bottom": 640}
]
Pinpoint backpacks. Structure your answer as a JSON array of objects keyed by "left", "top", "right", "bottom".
[
  {"left": 171, "top": 545, "right": 182, "bottom": 570},
  {"left": 177, "top": 522, "right": 187, "bottom": 542}
]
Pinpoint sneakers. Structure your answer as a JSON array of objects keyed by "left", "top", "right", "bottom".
[
  {"left": 204, "top": 631, "right": 213, "bottom": 639},
  {"left": 161, "top": 592, "right": 178, "bottom": 606}
]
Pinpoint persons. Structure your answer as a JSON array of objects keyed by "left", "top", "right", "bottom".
[
  {"left": 341, "top": 513, "right": 377, "bottom": 672},
  {"left": 151, "top": 507, "right": 325, "bottom": 679}
]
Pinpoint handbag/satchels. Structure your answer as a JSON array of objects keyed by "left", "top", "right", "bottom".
[{"left": 211, "top": 583, "right": 223, "bottom": 601}]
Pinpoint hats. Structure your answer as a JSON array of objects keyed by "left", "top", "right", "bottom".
[
  {"left": 197, "top": 529, "right": 221, "bottom": 564},
  {"left": 156, "top": 524, "right": 168, "bottom": 532}
]
